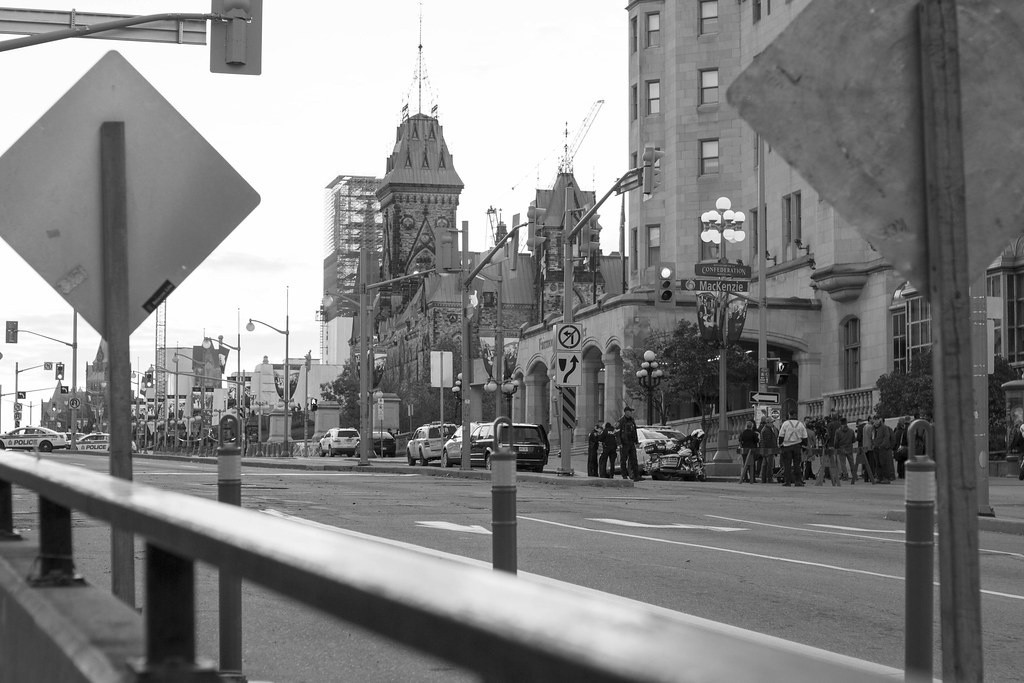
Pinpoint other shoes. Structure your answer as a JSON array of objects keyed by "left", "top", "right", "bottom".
[
  {"left": 630, "top": 475, "right": 645, "bottom": 480},
  {"left": 743, "top": 472, "right": 905, "bottom": 487}
]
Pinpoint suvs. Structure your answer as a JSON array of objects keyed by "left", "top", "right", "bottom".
[{"left": 318, "top": 427, "right": 361, "bottom": 457}]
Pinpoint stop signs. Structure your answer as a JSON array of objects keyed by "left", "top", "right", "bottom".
[{"left": 771, "top": 409, "right": 781, "bottom": 420}]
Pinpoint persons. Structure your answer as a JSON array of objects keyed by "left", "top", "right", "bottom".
[
  {"left": 828, "top": 412, "right": 934, "bottom": 484},
  {"left": 615, "top": 407, "right": 646, "bottom": 481},
  {"left": 1019, "top": 424, "right": 1024, "bottom": 480},
  {"left": 739, "top": 410, "right": 816, "bottom": 486},
  {"left": 588, "top": 422, "right": 616, "bottom": 477},
  {"left": 388, "top": 428, "right": 396, "bottom": 440}
]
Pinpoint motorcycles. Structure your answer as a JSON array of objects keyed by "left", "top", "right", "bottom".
[{"left": 644, "top": 443, "right": 707, "bottom": 482}]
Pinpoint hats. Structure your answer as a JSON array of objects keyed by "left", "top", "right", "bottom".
[
  {"left": 624, "top": 406, "right": 634, "bottom": 412},
  {"left": 840, "top": 418, "right": 847, "bottom": 423},
  {"left": 766, "top": 417, "right": 776, "bottom": 423}
]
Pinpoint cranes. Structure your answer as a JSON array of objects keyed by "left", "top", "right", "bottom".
[
  {"left": 549, "top": 100, "right": 605, "bottom": 187},
  {"left": 482, "top": 206, "right": 507, "bottom": 248}
]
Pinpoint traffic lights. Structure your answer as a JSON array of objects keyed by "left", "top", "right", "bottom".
[
  {"left": 311, "top": 398, "right": 317, "bottom": 411},
  {"left": 642, "top": 146, "right": 665, "bottom": 194},
  {"left": 774, "top": 361, "right": 792, "bottom": 386},
  {"left": 527, "top": 206, "right": 547, "bottom": 256},
  {"left": 580, "top": 204, "right": 602, "bottom": 259},
  {"left": 145, "top": 371, "right": 153, "bottom": 388},
  {"left": 55, "top": 364, "right": 64, "bottom": 380},
  {"left": 654, "top": 261, "right": 676, "bottom": 308}
]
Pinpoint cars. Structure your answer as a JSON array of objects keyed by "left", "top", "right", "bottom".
[
  {"left": 355, "top": 431, "right": 396, "bottom": 458},
  {"left": 597, "top": 426, "right": 687, "bottom": 477},
  {"left": 406, "top": 420, "right": 550, "bottom": 473},
  {"left": 0, "top": 426, "right": 137, "bottom": 454}
]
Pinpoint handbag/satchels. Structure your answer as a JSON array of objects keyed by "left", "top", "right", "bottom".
[{"left": 897, "top": 445, "right": 906, "bottom": 455}]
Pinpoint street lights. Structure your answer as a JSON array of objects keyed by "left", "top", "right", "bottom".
[
  {"left": 701, "top": 197, "right": 745, "bottom": 476},
  {"left": 173, "top": 328, "right": 206, "bottom": 456},
  {"left": 246, "top": 285, "right": 291, "bottom": 458},
  {"left": 149, "top": 341, "right": 179, "bottom": 455},
  {"left": 100, "top": 356, "right": 140, "bottom": 453},
  {"left": 201, "top": 308, "right": 240, "bottom": 447},
  {"left": 636, "top": 351, "right": 663, "bottom": 426}
]
